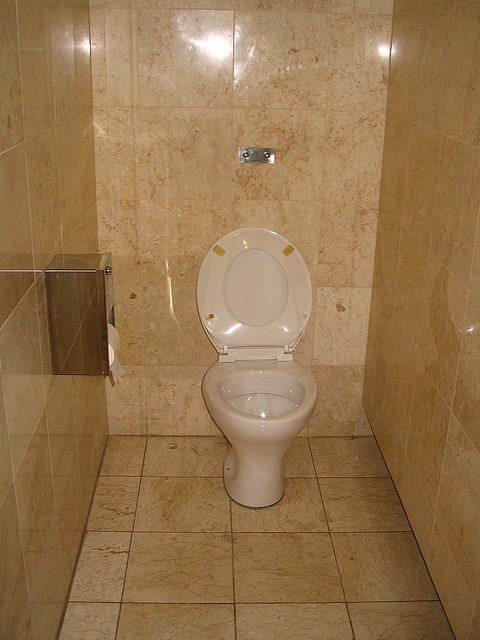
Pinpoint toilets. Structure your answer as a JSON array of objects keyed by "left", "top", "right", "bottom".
[{"left": 195, "top": 226, "right": 318, "bottom": 508}]
